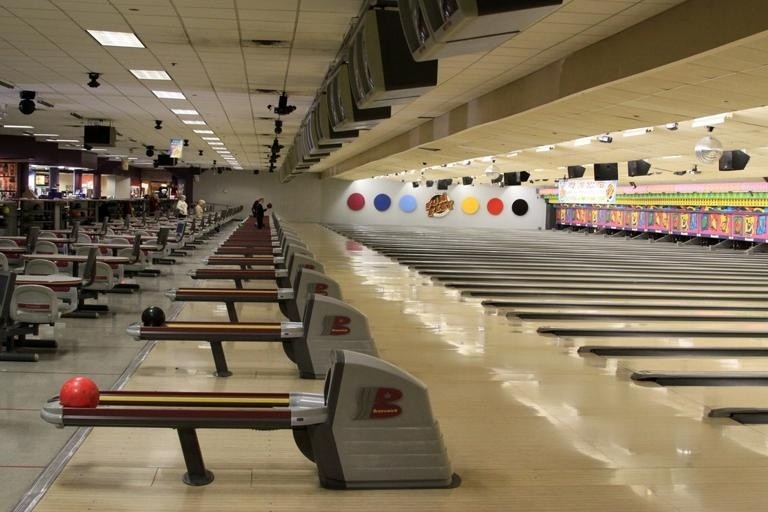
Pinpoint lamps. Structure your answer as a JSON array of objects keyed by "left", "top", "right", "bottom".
[
  {"left": 695, "top": 126, "right": 724, "bottom": 165},
  {"left": 485, "top": 160, "right": 500, "bottom": 180}
]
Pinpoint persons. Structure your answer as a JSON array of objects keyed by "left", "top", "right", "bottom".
[
  {"left": 252, "top": 197, "right": 260, "bottom": 217},
  {"left": 158, "top": 186, "right": 168, "bottom": 199},
  {"left": 144, "top": 194, "right": 150, "bottom": 204},
  {"left": 255, "top": 198, "right": 268, "bottom": 228},
  {"left": 150, "top": 196, "right": 157, "bottom": 212},
  {"left": 194, "top": 199, "right": 206, "bottom": 220},
  {"left": 176, "top": 195, "right": 188, "bottom": 220}
]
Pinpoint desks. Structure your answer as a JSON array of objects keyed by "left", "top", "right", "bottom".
[
  {"left": 0, "top": 275, "right": 84, "bottom": 362},
  {"left": 0, "top": 248, "right": 27, "bottom": 251},
  {"left": 103, "top": 235, "right": 176, "bottom": 277},
  {"left": 23, "top": 255, "right": 129, "bottom": 319},
  {"left": 69, "top": 243, "right": 158, "bottom": 293},
  {"left": 1, "top": 237, "right": 76, "bottom": 242}
]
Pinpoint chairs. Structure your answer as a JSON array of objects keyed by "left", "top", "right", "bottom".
[
  {"left": 26, "top": 226, "right": 40, "bottom": 252},
  {"left": 214, "top": 205, "right": 243, "bottom": 225},
  {"left": 200, "top": 216, "right": 204, "bottom": 231},
  {"left": 71, "top": 221, "right": 80, "bottom": 243},
  {"left": 190, "top": 219, "right": 196, "bottom": 235},
  {"left": 207, "top": 214, "right": 211, "bottom": 225},
  {"left": 39, "top": 231, "right": 57, "bottom": 239},
  {"left": 156, "top": 228, "right": 170, "bottom": 252},
  {"left": 174, "top": 209, "right": 180, "bottom": 220},
  {"left": 78, "top": 262, "right": 114, "bottom": 291},
  {"left": 141, "top": 212, "right": 147, "bottom": 228},
  {"left": 176, "top": 222, "right": 186, "bottom": 242},
  {"left": 25, "top": 259, "right": 58, "bottom": 275},
  {"left": 33, "top": 241, "right": 58, "bottom": 254},
  {"left": 101, "top": 217, "right": 109, "bottom": 235},
  {"left": 78, "top": 234, "right": 92, "bottom": 243},
  {"left": 0, "top": 239, "right": 18, "bottom": 247},
  {"left": 82, "top": 248, "right": 98, "bottom": 287},
  {"left": 130, "top": 235, "right": 141, "bottom": 265},
  {"left": 9, "top": 285, "right": 58, "bottom": 336},
  {"left": 166, "top": 210, "right": 170, "bottom": 220},
  {"left": 124, "top": 215, "right": 130, "bottom": 232},
  {"left": 154, "top": 211, "right": 159, "bottom": 223},
  {"left": 111, "top": 238, "right": 130, "bottom": 244}
]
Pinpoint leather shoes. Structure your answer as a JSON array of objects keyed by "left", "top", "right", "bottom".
[
  {"left": 262, "top": 224, "right": 265, "bottom": 227},
  {"left": 258, "top": 226, "right": 263, "bottom": 229}
]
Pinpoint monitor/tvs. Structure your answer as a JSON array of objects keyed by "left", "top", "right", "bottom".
[
  {"left": 348, "top": 9, "right": 438, "bottom": 110},
  {"left": 398, "top": 0, "right": 564, "bottom": 62},
  {"left": 279, "top": 94, "right": 359, "bottom": 184},
  {"left": 504, "top": 172, "right": 521, "bottom": 185},
  {"left": 325, "top": 65, "right": 391, "bottom": 132},
  {"left": 594, "top": 163, "right": 618, "bottom": 181}
]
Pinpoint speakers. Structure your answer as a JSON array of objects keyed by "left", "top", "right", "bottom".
[
  {"left": 628, "top": 160, "right": 651, "bottom": 177},
  {"left": 463, "top": 177, "right": 473, "bottom": 185},
  {"left": 568, "top": 166, "right": 586, "bottom": 179},
  {"left": 719, "top": 150, "right": 751, "bottom": 171}
]
